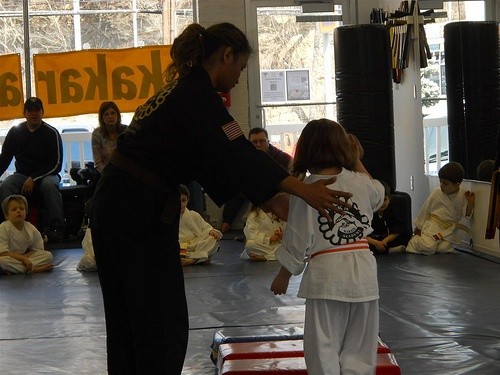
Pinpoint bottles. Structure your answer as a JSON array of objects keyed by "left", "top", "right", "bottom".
[{"left": 63, "top": 170, "right": 70, "bottom": 188}]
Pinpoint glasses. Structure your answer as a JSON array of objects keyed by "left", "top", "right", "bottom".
[{"left": 251, "top": 138, "right": 270, "bottom": 145}]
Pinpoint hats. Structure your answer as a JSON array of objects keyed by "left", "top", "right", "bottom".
[{"left": 23, "top": 97, "right": 44, "bottom": 109}]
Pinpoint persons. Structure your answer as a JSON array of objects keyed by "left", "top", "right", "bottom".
[
  {"left": 78, "top": 101, "right": 293, "bottom": 272},
  {"left": 271, "top": 118, "right": 386, "bottom": 375},
  {"left": 406, "top": 162, "right": 475, "bottom": 256},
  {"left": 0, "top": 194, "right": 54, "bottom": 274},
  {"left": 366, "top": 181, "right": 406, "bottom": 254},
  {"left": 0, "top": 97, "right": 69, "bottom": 240},
  {"left": 87, "top": 22, "right": 354, "bottom": 375},
  {"left": 477, "top": 160, "right": 497, "bottom": 182}
]
